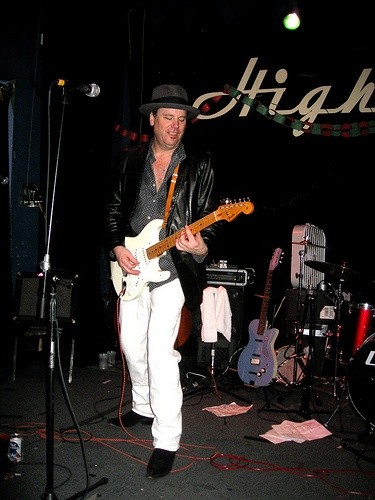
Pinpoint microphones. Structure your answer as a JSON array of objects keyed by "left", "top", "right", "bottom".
[{"left": 58, "top": 79, "right": 100, "bottom": 97}]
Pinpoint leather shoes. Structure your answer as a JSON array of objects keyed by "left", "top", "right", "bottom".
[
  {"left": 146, "top": 448, "right": 176, "bottom": 478},
  {"left": 107, "top": 410, "right": 155, "bottom": 426}
]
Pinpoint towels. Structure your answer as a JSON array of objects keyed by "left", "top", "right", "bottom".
[{"left": 199, "top": 287, "right": 235, "bottom": 342}]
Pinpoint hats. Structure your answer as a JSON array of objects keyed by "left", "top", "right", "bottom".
[{"left": 140, "top": 84, "right": 202, "bottom": 119}]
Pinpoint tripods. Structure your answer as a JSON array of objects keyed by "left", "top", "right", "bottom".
[
  {"left": 183, "top": 272, "right": 345, "bottom": 420},
  {"left": 29, "top": 98, "right": 108, "bottom": 500}
]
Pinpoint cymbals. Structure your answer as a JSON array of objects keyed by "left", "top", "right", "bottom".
[{"left": 301, "top": 260, "right": 352, "bottom": 278}]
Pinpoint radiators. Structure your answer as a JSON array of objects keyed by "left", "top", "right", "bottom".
[{"left": 290, "top": 223, "right": 326, "bottom": 289}]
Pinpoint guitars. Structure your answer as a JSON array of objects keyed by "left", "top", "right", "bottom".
[
  {"left": 110, "top": 196, "right": 256, "bottom": 303},
  {"left": 237, "top": 247, "right": 285, "bottom": 387}
]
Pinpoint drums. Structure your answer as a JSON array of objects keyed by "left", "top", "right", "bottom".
[
  {"left": 346, "top": 332, "right": 375, "bottom": 430},
  {"left": 324, "top": 296, "right": 375, "bottom": 364},
  {"left": 276, "top": 345, "right": 308, "bottom": 385}
]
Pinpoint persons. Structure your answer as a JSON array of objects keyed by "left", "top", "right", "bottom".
[{"left": 104, "top": 82, "right": 220, "bottom": 479}]
